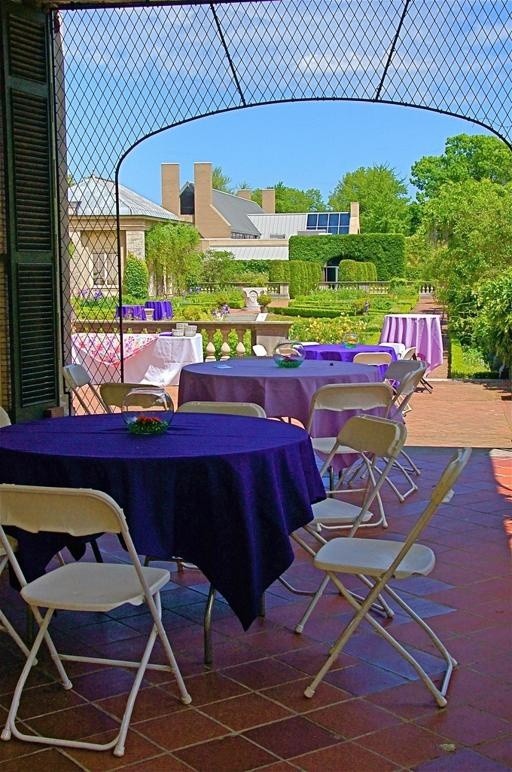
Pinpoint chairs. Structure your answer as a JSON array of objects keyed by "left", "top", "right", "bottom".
[
  {"left": 304, "top": 445, "right": 472, "bottom": 706},
  {"left": 0, "top": 342, "right": 451, "bottom": 669},
  {"left": 3, "top": 484, "right": 192, "bottom": 755}
]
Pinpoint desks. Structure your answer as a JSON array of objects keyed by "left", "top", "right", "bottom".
[
  {"left": 67, "top": 331, "right": 205, "bottom": 388},
  {"left": 146, "top": 301, "right": 173, "bottom": 320},
  {"left": 116, "top": 306, "right": 145, "bottom": 320},
  {"left": 380, "top": 314, "right": 443, "bottom": 376}
]
